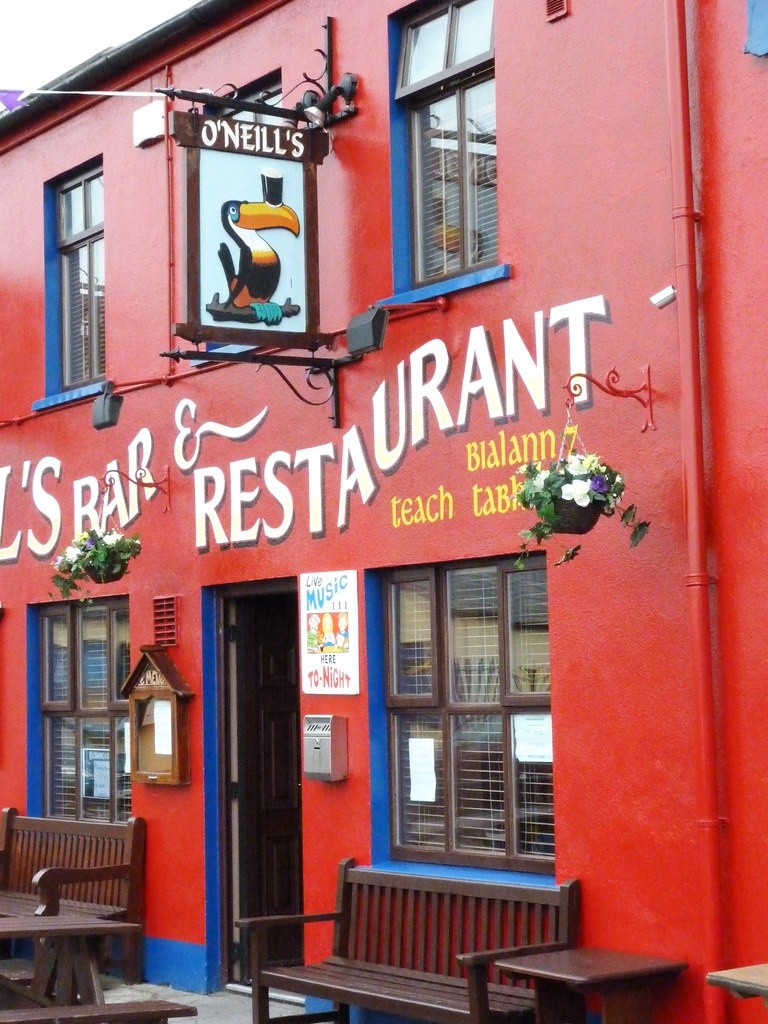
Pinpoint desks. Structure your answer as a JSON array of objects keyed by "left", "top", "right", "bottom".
[
  {"left": 493, "top": 946, "right": 693, "bottom": 1024},
  {"left": 704, "top": 964, "right": 768, "bottom": 1007},
  {"left": 0, "top": 914, "right": 148, "bottom": 1004}
]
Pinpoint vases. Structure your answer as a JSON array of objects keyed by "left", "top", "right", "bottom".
[
  {"left": 86, "top": 558, "right": 127, "bottom": 583},
  {"left": 533, "top": 491, "right": 610, "bottom": 534}
]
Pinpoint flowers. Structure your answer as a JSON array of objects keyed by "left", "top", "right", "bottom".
[
  {"left": 505, "top": 447, "right": 652, "bottom": 573},
  {"left": 46, "top": 530, "right": 149, "bottom": 605}
]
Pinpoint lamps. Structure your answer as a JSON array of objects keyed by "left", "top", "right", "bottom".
[
  {"left": 91, "top": 373, "right": 174, "bottom": 430},
  {"left": 345, "top": 296, "right": 448, "bottom": 359},
  {"left": 302, "top": 72, "right": 359, "bottom": 129}
]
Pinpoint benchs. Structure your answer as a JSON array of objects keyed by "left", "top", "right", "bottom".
[
  {"left": 0, "top": 998, "right": 201, "bottom": 1024},
  {"left": 232, "top": 856, "right": 585, "bottom": 1024},
  {"left": 0, "top": 806, "right": 149, "bottom": 987}
]
